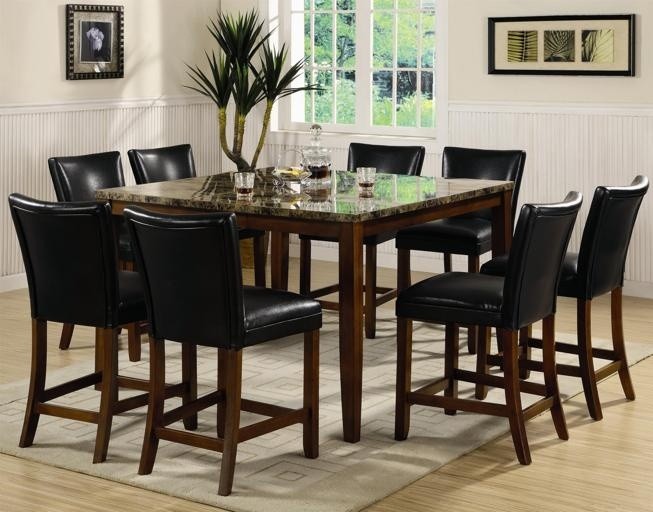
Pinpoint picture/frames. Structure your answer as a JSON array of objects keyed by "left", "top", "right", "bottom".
[
  {"left": 65, "top": 5, "right": 124, "bottom": 79},
  {"left": 487, "top": 13, "right": 635, "bottom": 77}
]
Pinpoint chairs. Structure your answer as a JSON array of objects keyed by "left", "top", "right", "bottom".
[
  {"left": 299, "top": 142, "right": 424, "bottom": 338},
  {"left": 8, "top": 192, "right": 197, "bottom": 464},
  {"left": 476, "top": 174, "right": 649, "bottom": 420},
  {"left": 124, "top": 204, "right": 322, "bottom": 496},
  {"left": 127, "top": 144, "right": 270, "bottom": 287},
  {"left": 393, "top": 191, "right": 583, "bottom": 465},
  {"left": 396, "top": 146, "right": 526, "bottom": 352},
  {"left": 48, "top": 151, "right": 134, "bottom": 272}
]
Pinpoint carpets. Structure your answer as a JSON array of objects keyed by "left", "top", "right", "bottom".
[{"left": 0, "top": 308, "right": 653, "bottom": 512}]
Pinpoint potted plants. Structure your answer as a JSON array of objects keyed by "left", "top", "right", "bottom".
[{"left": 180, "top": 9, "right": 326, "bottom": 270}]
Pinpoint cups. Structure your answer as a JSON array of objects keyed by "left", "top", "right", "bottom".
[
  {"left": 235, "top": 172, "right": 254, "bottom": 196},
  {"left": 235, "top": 197, "right": 253, "bottom": 207},
  {"left": 356, "top": 167, "right": 375, "bottom": 196}
]
